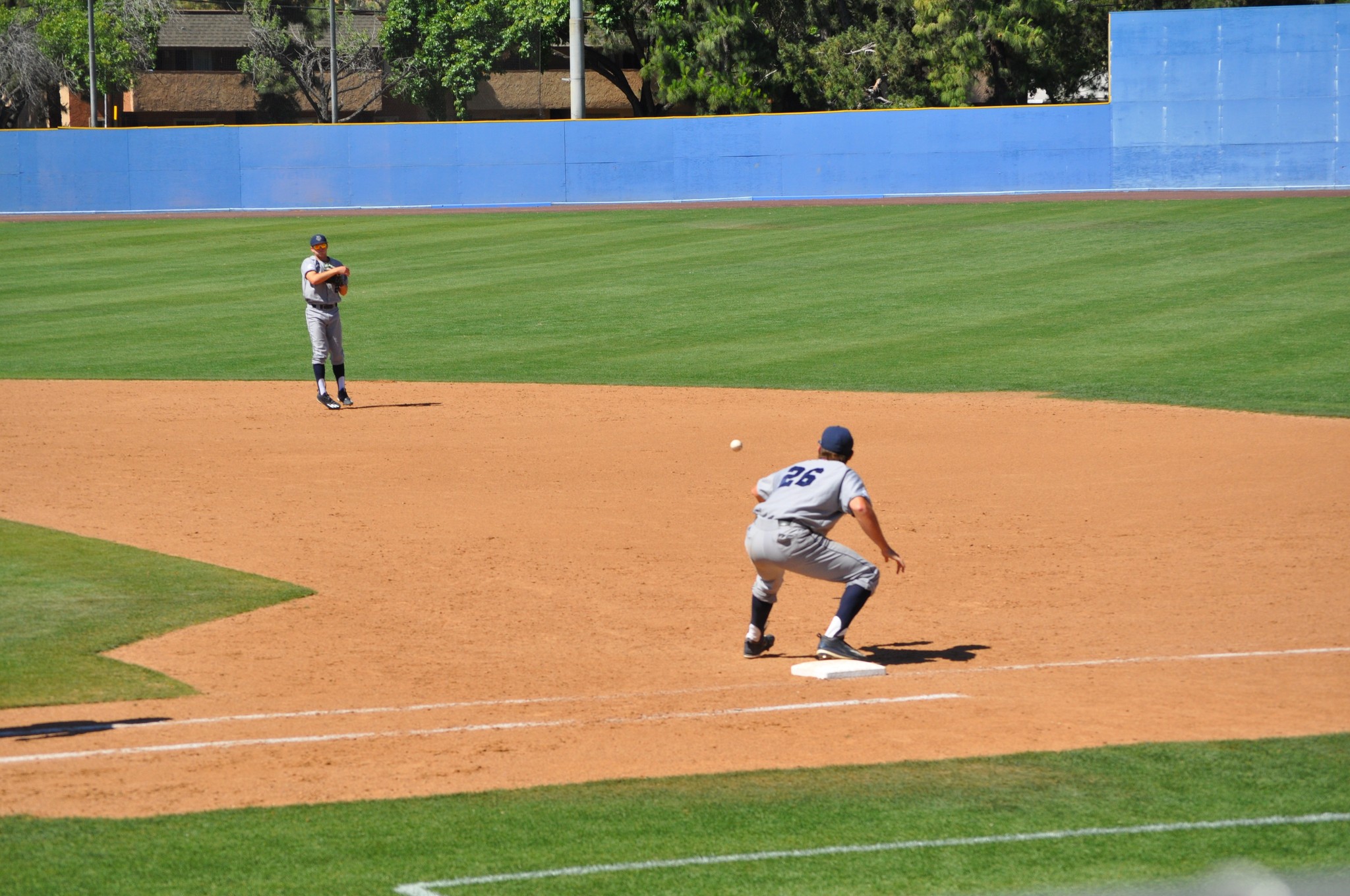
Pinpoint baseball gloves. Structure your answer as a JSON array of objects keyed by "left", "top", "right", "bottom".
[{"left": 323, "top": 263, "right": 343, "bottom": 287}]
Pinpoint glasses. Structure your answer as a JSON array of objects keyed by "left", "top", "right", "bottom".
[{"left": 312, "top": 243, "right": 327, "bottom": 249}]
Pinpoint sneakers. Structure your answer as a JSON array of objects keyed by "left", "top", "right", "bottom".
[
  {"left": 742, "top": 633, "right": 775, "bottom": 659},
  {"left": 336, "top": 387, "right": 353, "bottom": 406},
  {"left": 816, "top": 633, "right": 871, "bottom": 663},
  {"left": 316, "top": 389, "right": 341, "bottom": 410}
]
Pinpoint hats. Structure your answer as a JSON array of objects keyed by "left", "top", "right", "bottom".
[
  {"left": 818, "top": 425, "right": 853, "bottom": 457},
  {"left": 310, "top": 233, "right": 327, "bottom": 247}
]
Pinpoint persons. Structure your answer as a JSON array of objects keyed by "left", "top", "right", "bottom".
[
  {"left": 744, "top": 426, "right": 906, "bottom": 660},
  {"left": 301, "top": 235, "right": 353, "bottom": 409}
]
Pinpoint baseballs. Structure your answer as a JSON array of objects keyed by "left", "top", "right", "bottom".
[{"left": 730, "top": 440, "right": 742, "bottom": 452}]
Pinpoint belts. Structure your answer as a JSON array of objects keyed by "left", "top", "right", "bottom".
[
  {"left": 775, "top": 517, "right": 815, "bottom": 534},
  {"left": 311, "top": 302, "right": 337, "bottom": 310}
]
